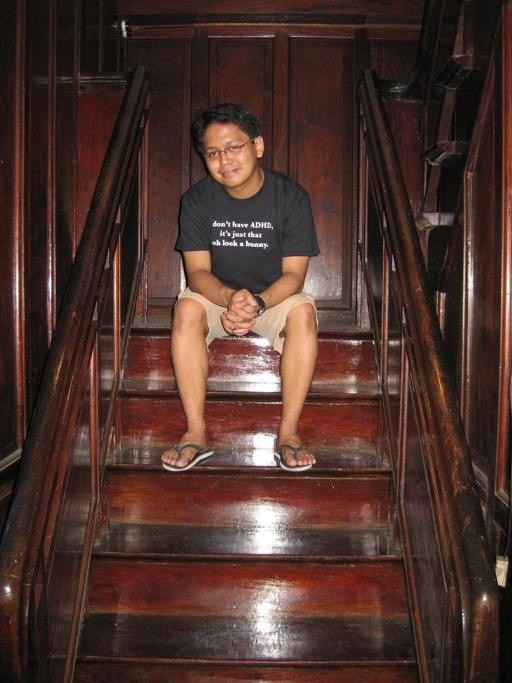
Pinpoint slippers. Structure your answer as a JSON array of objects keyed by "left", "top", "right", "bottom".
[
  {"left": 160, "top": 443, "right": 214, "bottom": 471},
  {"left": 274, "top": 444, "right": 317, "bottom": 473}
]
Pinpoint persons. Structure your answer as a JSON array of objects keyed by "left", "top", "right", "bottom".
[{"left": 161, "top": 104, "right": 320, "bottom": 473}]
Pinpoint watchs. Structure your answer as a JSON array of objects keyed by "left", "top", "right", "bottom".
[{"left": 252, "top": 294, "right": 265, "bottom": 316}]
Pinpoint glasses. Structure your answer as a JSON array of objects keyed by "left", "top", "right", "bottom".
[{"left": 204, "top": 136, "right": 256, "bottom": 159}]
